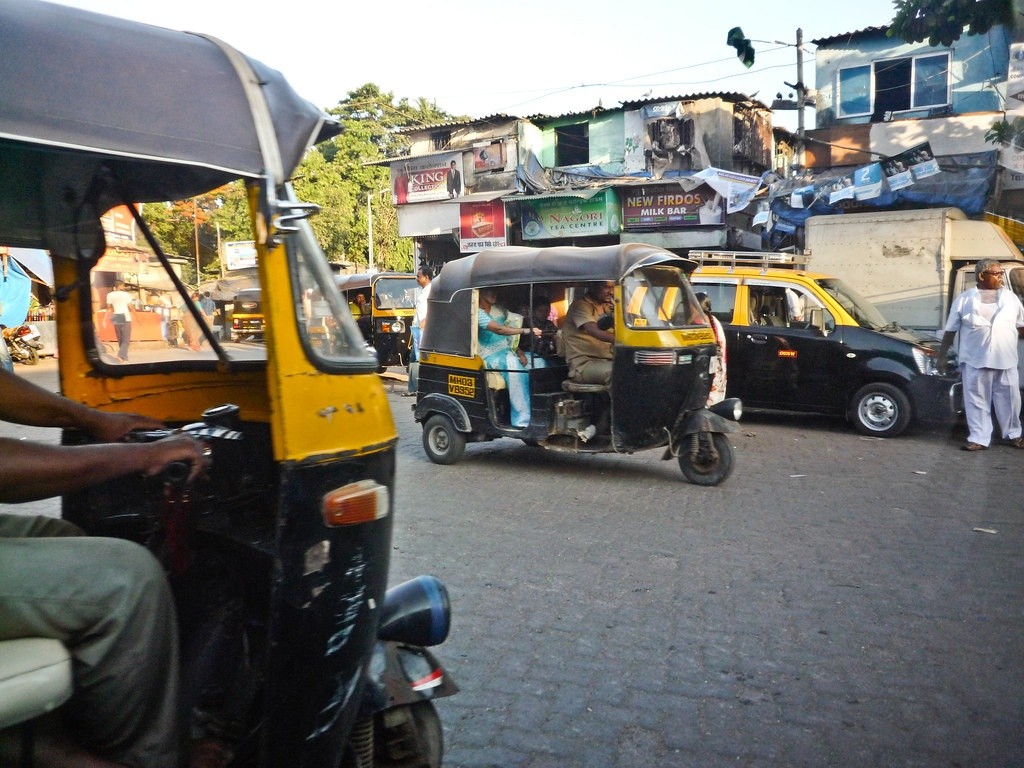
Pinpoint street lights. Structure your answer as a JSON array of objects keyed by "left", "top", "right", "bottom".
[{"left": 725, "top": 26, "right": 806, "bottom": 174}]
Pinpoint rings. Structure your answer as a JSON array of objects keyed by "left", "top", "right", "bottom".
[{"left": 203, "top": 448, "right": 213, "bottom": 457}]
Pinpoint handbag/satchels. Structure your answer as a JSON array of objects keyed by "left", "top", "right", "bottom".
[
  {"left": 111, "top": 313, "right": 126, "bottom": 326},
  {"left": 504, "top": 311, "right": 524, "bottom": 354}
]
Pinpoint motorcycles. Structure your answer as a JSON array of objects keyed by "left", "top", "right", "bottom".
[
  {"left": 231, "top": 288, "right": 270, "bottom": 343},
  {"left": 0, "top": 1, "right": 456, "bottom": 767},
  {"left": 309, "top": 273, "right": 424, "bottom": 367},
  {"left": 414, "top": 242, "right": 744, "bottom": 483}
]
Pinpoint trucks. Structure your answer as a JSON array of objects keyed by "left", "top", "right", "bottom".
[{"left": 799, "top": 209, "right": 1024, "bottom": 392}]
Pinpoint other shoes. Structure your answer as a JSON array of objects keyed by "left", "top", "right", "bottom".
[
  {"left": 963, "top": 443, "right": 983, "bottom": 451},
  {"left": 1000, "top": 438, "right": 1024, "bottom": 449},
  {"left": 401, "top": 392, "right": 416, "bottom": 396}
]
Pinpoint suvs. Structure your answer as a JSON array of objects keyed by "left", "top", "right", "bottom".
[{"left": 623, "top": 251, "right": 969, "bottom": 442}]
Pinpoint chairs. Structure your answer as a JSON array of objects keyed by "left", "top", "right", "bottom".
[
  {"left": 748, "top": 296, "right": 786, "bottom": 329},
  {"left": 555, "top": 328, "right": 608, "bottom": 392},
  {"left": 0, "top": 636, "right": 75, "bottom": 768}
]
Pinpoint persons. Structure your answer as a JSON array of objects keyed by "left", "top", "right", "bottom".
[
  {"left": 786, "top": 287, "right": 804, "bottom": 322},
  {"left": 476, "top": 288, "right": 542, "bottom": 427},
  {"left": 886, "top": 160, "right": 907, "bottom": 177},
  {"left": 558, "top": 281, "right": 616, "bottom": 406},
  {"left": 179, "top": 290, "right": 221, "bottom": 351},
  {"left": 391, "top": 287, "right": 406, "bottom": 307},
  {"left": 446, "top": 161, "right": 461, "bottom": 199},
  {"left": 353, "top": 292, "right": 369, "bottom": 323},
  {"left": 910, "top": 148, "right": 930, "bottom": 163},
  {"left": 547, "top": 305, "right": 558, "bottom": 327},
  {"left": 831, "top": 176, "right": 853, "bottom": 191},
  {"left": 106, "top": 280, "right": 136, "bottom": 361},
  {"left": 394, "top": 168, "right": 408, "bottom": 205},
  {"left": 938, "top": 258, "right": 1024, "bottom": 450},
  {"left": 0, "top": 329, "right": 14, "bottom": 373},
  {"left": 523, "top": 298, "right": 559, "bottom": 356},
  {"left": 0, "top": 365, "right": 211, "bottom": 768},
  {"left": 693, "top": 292, "right": 729, "bottom": 409},
  {"left": 401, "top": 265, "right": 433, "bottom": 395}
]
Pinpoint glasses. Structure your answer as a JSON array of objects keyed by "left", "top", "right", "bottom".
[{"left": 983, "top": 270, "right": 1004, "bottom": 276}]
